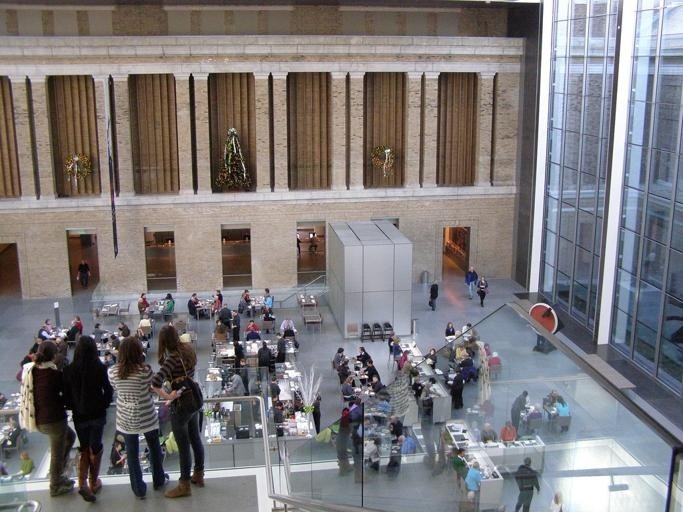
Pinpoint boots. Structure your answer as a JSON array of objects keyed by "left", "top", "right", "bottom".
[
  {"left": 77, "top": 446, "right": 97, "bottom": 502},
  {"left": 339, "top": 456, "right": 353, "bottom": 475},
  {"left": 89, "top": 445, "right": 102, "bottom": 494},
  {"left": 190, "top": 470, "right": 204, "bottom": 487},
  {"left": 353, "top": 463, "right": 368, "bottom": 483},
  {"left": 165, "top": 480, "right": 192, "bottom": 498}
]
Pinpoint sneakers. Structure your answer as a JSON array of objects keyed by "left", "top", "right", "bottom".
[
  {"left": 50, "top": 479, "right": 75, "bottom": 497},
  {"left": 156, "top": 473, "right": 169, "bottom": 490}
]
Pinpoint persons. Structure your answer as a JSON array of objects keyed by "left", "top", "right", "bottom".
[
  {"left": 550, "top": 491, "right": 566, "bottom": 512},
  {"left": 451, "top": 447, "right": 468, "bottom": 478},
  {"left": 61, "top": 334, "right": 113, "bottom": 501},
  {"left": 106, "top": 335, "right": 169, "bottom": 499},
  {"left": 15, "top": 452, "right": 32, "bottom": 475},
  {"left": 107, "top": 431, "right": 128, "bottom": 475},
  {"left": 464, "top": 265, "right": 478, "bottom": 299},
  {"left": 331, "top": 346, "right": 416, "bottom": 455},
  {"left": 15, "top": 339, "right": 75, "bottom": 497},
  {"left": 149, "top": 325, "right": 204, "bottom": 497},
  {"left": 514, "top": 456, "right": 539, "bottom": 511},
  {"left": 296, "top": 236, "right": 301, "bottom": 255},
  {"left": 476, "top": 274, "right": 488, "bottom": 307},
  {"left": 307, "top": 231, "right": 320, "bottom": 252},
  {"left": 75, "top": 259, "right": 90, "bottom": 290},
  {"left": 94, "top": 321, "right": 148, "bottom": 364},
  {"left": 238, "top": 288, "right": 273, "bottom": 317},
  {"left": 389, "top": 322, "right": 490, "bottom": 408},
  {"left": 312, "top": 393, "right": 321, "bottom": 435},
  {"left": 464, "top": 461, "right": 486, "bottom": 494},
  {"left": 39, "top": 316, "right": 83, "bottom": 349},
  {"left": 472, "top": 388, "right": 571, "bottom": 446},
  {"left": 188, "top": 290, "right": 223, "bottom": 319},
  {"left": 138, "top": 293, "right": 174, "bottom": 321},
  {"left": 427, "top": 279, "right": 438, "bottom": 311},
  {"left": 212, "top": 302, "right": 304, "bottom": 439}
]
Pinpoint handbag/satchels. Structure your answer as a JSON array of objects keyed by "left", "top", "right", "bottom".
[{"left": 168, "top": 375, "right": 204, "bottom": 418}]
